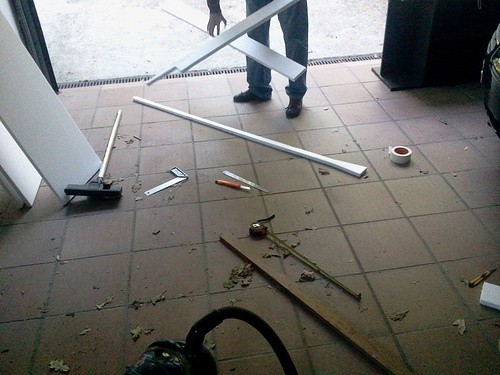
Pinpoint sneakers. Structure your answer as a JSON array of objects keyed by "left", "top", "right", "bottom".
[
  {"left": 286, "top": 98, "right": 303, "bottom": 118},
  {"left": 234, "top": 91, "right": 271, "bottom": 102}
]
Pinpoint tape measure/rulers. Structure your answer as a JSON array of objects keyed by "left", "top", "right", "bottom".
[
  {"left": 249, "top": 214, "right": 361, "bottom": 299},
  {"left": 222, "top": 170, "right": 270, "bottom": 193}
]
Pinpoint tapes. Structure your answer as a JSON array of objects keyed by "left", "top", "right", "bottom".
[{"left": 390, "top": 145, "right": 412, "bottom": 164}]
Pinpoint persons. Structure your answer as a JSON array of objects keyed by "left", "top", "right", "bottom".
[{"left": 205, "top": 0, "right": 312, "bottom": 119}]
